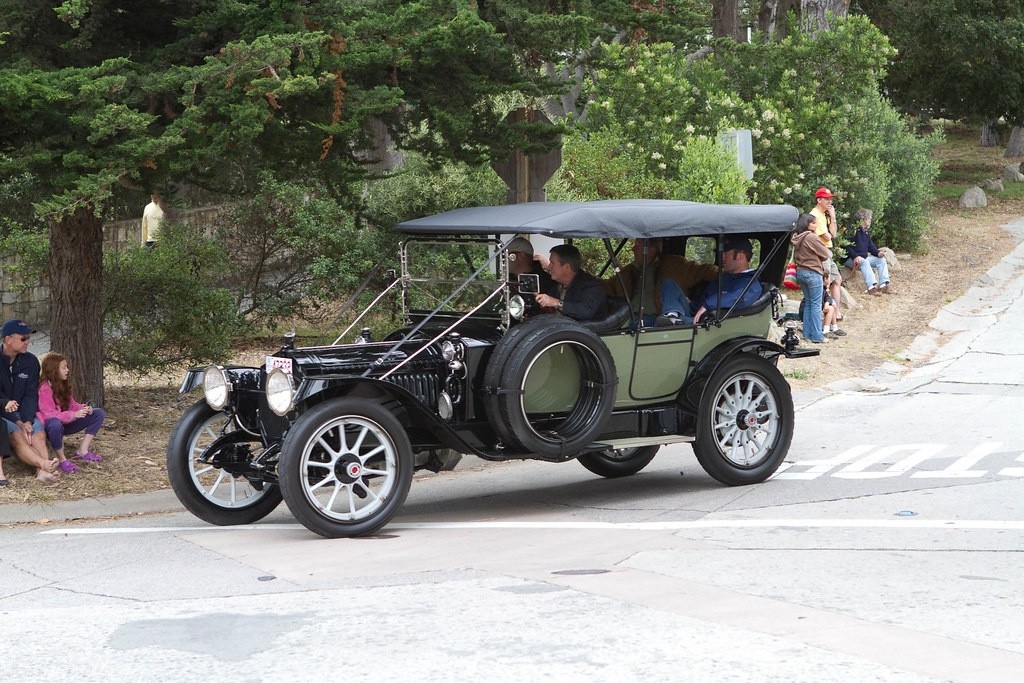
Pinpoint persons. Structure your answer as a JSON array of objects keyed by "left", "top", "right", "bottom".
[
  {"left": 807, "top": 190, "right": 845, "bottom": 321},
  {"left": 0, "top": 319, "right": 61, "bottom": 486},
  {"left": 628, "top": 237, "right": 767, "bottom": 330},
  {"left": 797, "top": 269, "right": 846, "bottom": 340},
  {"left": 594, "top": 238, "right": 729, "bottom": 329},
  {"left": 484, "top": 236, "right": 560, "bottom": 315},
  {"left": 534, "top": 244, "right": 609, "bottom": 322},
  {"left": 790, "top": 212, "right": 836, "bottom": 344},
  {"left": 839, "top": 207, "right": 890, "bottom": 294},
  {"left": 36, "top": 351, "right": 105, "bottom": 472},
  {"left": 141, "top": 190, "right": 164, "bottom": 249}
]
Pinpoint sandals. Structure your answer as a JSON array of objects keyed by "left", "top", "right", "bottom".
[
  {"left": 58, "top": 458, "right": 79, "bottom": 474},
  {"left": 75, "top": 449, "right": 103, "bottom": 463}
]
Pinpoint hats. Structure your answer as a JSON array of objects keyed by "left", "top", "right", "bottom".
[
  {"left": 713, "top": 238, "right": 753, "bottom": 252},
  {"left": 494, "top": 236, "right": 534, "bottom": 256},
  {"left": 0, "top": 319, "right": 37, "bottom": 338},
  {"left": 815, "top": 187, "right": 836, "bottom": 198}
]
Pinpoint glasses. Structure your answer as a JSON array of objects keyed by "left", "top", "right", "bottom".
[{"left": 10, "top": 335, "right": 30, "bottom": 342}]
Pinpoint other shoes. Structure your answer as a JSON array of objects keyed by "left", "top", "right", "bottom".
[
  {"left": 0, "top": 480, "right": 10, "bottom": 485},
  {"left": 867, "top": 287, "right": 882, "bottom": 296},
  {"left": 878, "top": 286, "right": 896, "bottom": 294},
  {"left": 822, "top": 331, "right": 838, "bottom": 339},
  {"left": 831, "top": 328, "right": 847, "bottom": 336},
  {"left": 655, "top": 311, "right": 684, "bottom": 327},
  {"left": 821, "top": 337, "right": 830, "bottom": 343}
]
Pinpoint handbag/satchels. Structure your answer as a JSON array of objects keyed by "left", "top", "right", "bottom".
[{"left": 782, "top": 247, "right": 801, "bottom": 290}]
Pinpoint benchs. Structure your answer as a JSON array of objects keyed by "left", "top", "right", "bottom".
[
  {"left": 693, "top": 280, "right": 778, "bottom": 325},
  {"left": 580, "top": 294, "right": 633, "bottom": 333}
]
